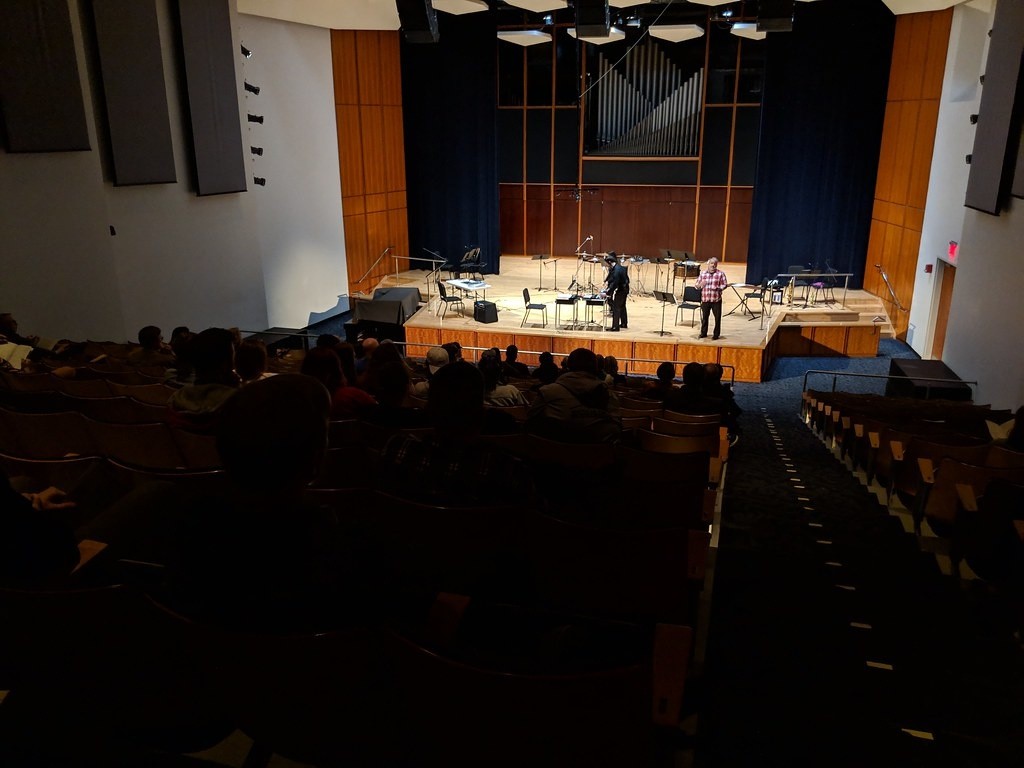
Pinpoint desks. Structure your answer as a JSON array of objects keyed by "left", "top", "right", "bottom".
[
  {"left": 778, "top": 274, "right": 854, "bottom": 309},
  {"left": 722, "top": 283, "right": 765, "bottom": 321},
  {"left": 554, "top": 298, "right": 578, "bottom": 331},
  {"left": 446, "top": 278, "right": 493, "bottom": 311},
  {"left": 672, "top": 276, "right": 698, "bottom": 297},
  {"left": 584, "top": 299, "right": 607, "bottom": 331}
]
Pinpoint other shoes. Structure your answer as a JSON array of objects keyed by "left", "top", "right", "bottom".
[
  {"left": 605, "top": 327, "right": 619, "bottom": 332},
  {"left": 619, "top": 324, "right": 627, "bottom": 328},
  {"left": 711, "top": 335, "right": 719, "bottom": 340},
  {"left": 699, "top": 334, "right": 707, "bottom": 338}
]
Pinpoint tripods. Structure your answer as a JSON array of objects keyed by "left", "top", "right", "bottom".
[
  {"left": 631, "top": 259, "right": 646, "bottom": 294},
  {"left": 653, "top": 291, "right": 676, "bottom": 337},
  {"left": 531, "top": 255, "right": 565, "bottom": 294}
]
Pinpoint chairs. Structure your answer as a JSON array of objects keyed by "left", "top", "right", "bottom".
[
  {"left": 0, "top": 339, "right": 732, "bottom": 768},
  {"left": 674, "top": 286, "right": 702, "bottom": 328},
  {"left": 741, "top": 266, "right": 838, "bottom": 317},
  {"left": 800, "top": 388, "right": 1024, "bottom": 654},
  {"left": 520, "top": 288, "right": 548, "bottom": 329},
  {"left": 436, "top": 281, "right": 466, "bottom": 322},
  {"left": 740, "top": 276, "right": 770, "bottom": 317}
]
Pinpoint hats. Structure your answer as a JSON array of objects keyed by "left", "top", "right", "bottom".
[{"left": 426, "top": 348, "right": 449, "bottom": 374}]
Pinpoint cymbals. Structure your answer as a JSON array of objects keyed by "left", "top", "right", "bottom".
[
  {"left": 595, "top": 253, "right": 609, "bottom": 256},
  {"left": 616, "top": 255, "right": 631, "bottom": 258},
  {"left": 587, "top": 258, "right": 601, "bottom": 262},
  {"left": 574, "top": 251, "right": 594, "bottom": 257}
]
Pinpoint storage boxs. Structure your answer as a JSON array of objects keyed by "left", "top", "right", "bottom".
[{"left": 674, "top": 265, "right": 701, "bottom": 277}]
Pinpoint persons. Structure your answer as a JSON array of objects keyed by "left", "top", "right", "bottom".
[
  {"left": 988, "top": 406, "right": 1024, "bottom": 453},
  {"left": 0, "top": 313, "right": 738, "bottom": 634},
  {"left": 602, "top": 251, "right": 630, "bottom": 332},
  {"left": 694, "top": 257, "right": 727, "bottom": 340}
]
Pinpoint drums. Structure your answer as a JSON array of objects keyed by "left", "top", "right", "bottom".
[{"left": 673, "top": 259, "right": 701, "bottom": 279}]
